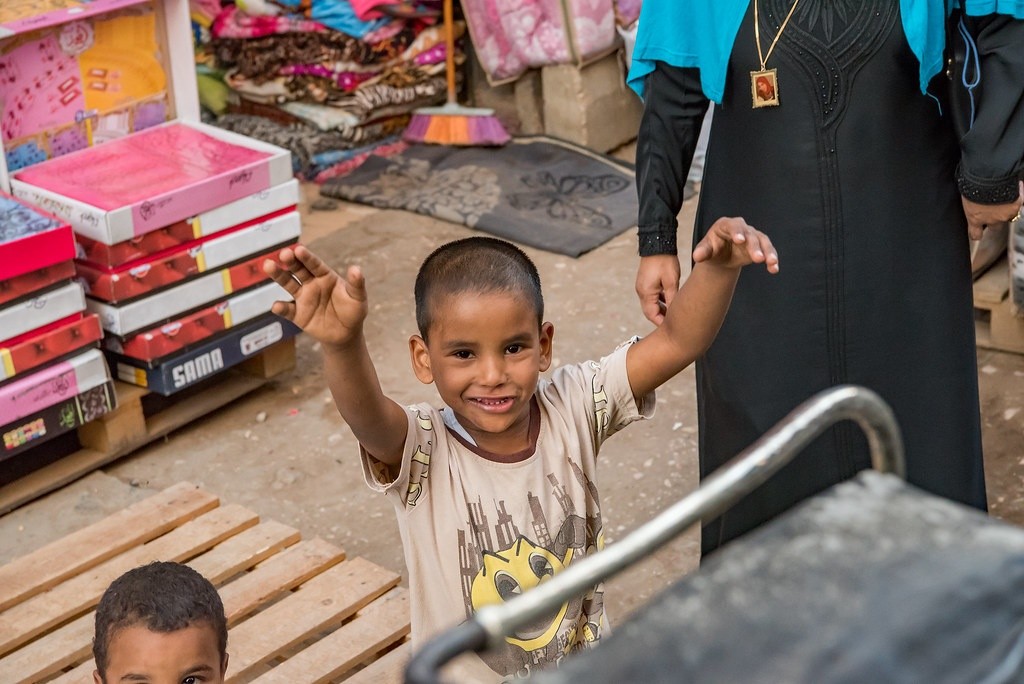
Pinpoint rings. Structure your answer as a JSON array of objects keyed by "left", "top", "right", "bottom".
[{"left": 1009, "top": 212, "right": 1021, "bottom": 222}]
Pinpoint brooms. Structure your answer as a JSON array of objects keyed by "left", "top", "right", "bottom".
[{"left": 402, "top": 1, "right": 513, "bottom": 148}]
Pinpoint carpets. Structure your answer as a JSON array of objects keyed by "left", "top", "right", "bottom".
[{"left": 319, "top": 132, "right": 698, "bottom": 259}]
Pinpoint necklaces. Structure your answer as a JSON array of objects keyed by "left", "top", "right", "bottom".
[{"left": 749, "top": 0, "right": 798, "bottom": 108}]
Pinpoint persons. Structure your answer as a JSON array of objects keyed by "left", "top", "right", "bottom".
[
  {"left": 262, "top": 216, "right": 779, "bottom": 684},
  {"left": 93, "top": 562, "right": 230, "bottom": 684},
  {"left": 756, "top": 77, "right": 775, "bottom": 102},
  {"left": 625, "top": 0, "right": 1024, "bottom": 572}
]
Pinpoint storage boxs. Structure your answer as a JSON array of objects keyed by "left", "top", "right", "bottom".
[{"left": 0, "top": 0, "right": 303, "bottom": 469}]
